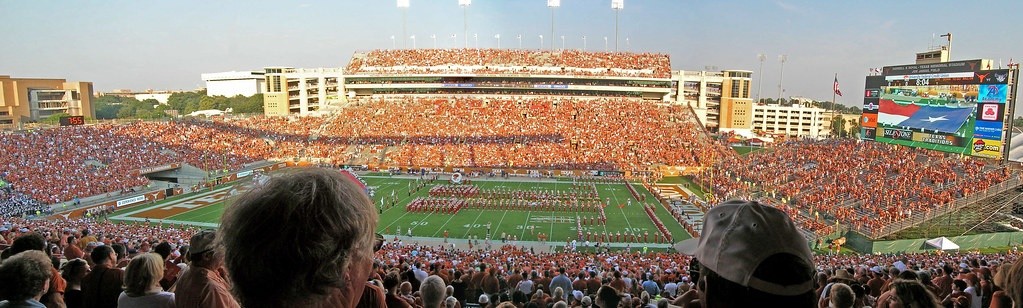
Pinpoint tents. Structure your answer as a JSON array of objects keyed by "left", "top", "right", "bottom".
[{"left": 924, "top": 236, "right": 959, "bottom": 253}]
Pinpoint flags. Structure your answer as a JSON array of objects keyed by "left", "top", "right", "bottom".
[{"left": 834, "top": 77, "right": 842, "bottom": 96}]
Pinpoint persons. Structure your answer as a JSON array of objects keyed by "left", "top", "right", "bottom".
[
  {"left": 81, "top": 229, "right": 97, "bottom": 247},
  {"left": 936, "top": 264, "right": 954, "bottom": 297},
  {"left": 549, "top": 267, "right": 573, "bottom": 300},
  {"left": 868, "top": 266, "right": 884, "bottom": 298},
  {"left": 974, "top": 267, "right": 992, "bottom": 308},
  {"left": 643, "top": 245, "right": 647, "bottom": 254},
  {"left": 0, "top": 46, "right": 1023, "bottom": 308},
  {"left": 610, "top": 271, "right": 626, "bottom": 292},
  {"left": 65, "top": 236, "right": 85, "bottom": 261},
  {"left": 516, "top": 271, "right": 535, "bottom": 300},
  {"left": 679, "top": 277, "right": 689, "bottom": 291},
  {"left": 414, "top": 262, "right": 428, "bottom": 282},
  {"left": 487, "top": 222, "right": 491, "bottom": 234},
  {"left": 587, "top": 271, "right": 601, "bottom": 294},
  {"left": 642, "top": 275, "right": 660, "bottom": 297},
  {"left": 212, "top": 166, "right": 376, "bottom": 308},
  {"left": 420, "top": 275, "right": 445, "bottom": 308},
  {"left": 667, "top": 200, "right": 819, "bottom": 308},
  {"left": 480, "top": 267, "right": 499, "bottom": 302}
]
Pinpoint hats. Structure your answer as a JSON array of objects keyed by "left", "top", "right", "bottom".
[
  {"left": 682, "top": 277, "right": 688, "bottom": 282},
  {"left": 581, "top": 296, "right": 591, "bottom": 306},
  {"left": 573, "top": 290, "right": 583, "bottom": 301},
  {"left": 674, "top": 200, "right": 818, "bottom": 296},
  {"left": 478, "top": 294, "right": 489, "bottom": 303},
  {"left": 622, "top": 271, "right": 628, "bottom": 275},
  {"left": 665, "top": 269, "right": 671, "bottom": 273},
  {"left": 832, "top": 269, "right": 855, "bottom": 282},
  {"left": 0, "top": 227, "right": 8, "bottom": 231},
  {"left": 188, "top": 231, "right": 225, "bottom": 257}
]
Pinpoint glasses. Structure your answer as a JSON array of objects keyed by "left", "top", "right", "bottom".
[
  {"left": 373, "top": 233, "right": 386, "bottom": 252},
  {"left": 689, "top": 257, "right": 704, "bottom": 285},
  {"left": 114, "top": 253, "right": 118, "bottom": 256}
]
